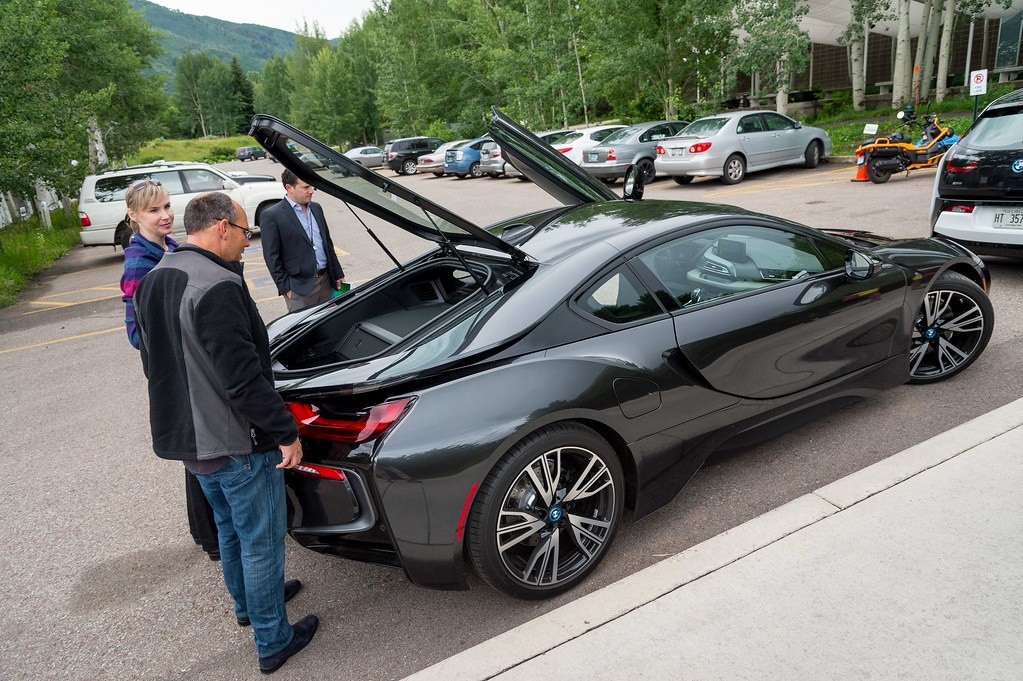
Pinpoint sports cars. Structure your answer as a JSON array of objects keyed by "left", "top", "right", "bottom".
[{"left": 236, "top": 108, "right": 995, "bottom": 603}]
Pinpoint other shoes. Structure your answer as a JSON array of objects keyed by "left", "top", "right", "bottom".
[{"left": 208, "top": 550, "right": 221, "bottom": 561}]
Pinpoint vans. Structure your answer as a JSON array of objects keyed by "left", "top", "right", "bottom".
[{"left": 237, "top": 146, "right": 267, "bottom": 162}]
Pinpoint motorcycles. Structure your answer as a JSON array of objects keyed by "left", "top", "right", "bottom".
[{"left": 861, "top": 100, "right": 955, "bottom": 184}]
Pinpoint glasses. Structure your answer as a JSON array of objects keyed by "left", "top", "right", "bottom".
[
  {"left": 127, "top": 179, "right": 162, "bottom": 208},
  {"left": 213, "top": 217, "right": 253, "bottom": 239}
]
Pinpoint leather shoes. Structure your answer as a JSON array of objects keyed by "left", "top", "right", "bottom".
[
  {"left": 259, "top": 613, "right": 318, "bottom": 674},
  {"left": 237, "top": 579, "right": 302, "bottom": 626}
]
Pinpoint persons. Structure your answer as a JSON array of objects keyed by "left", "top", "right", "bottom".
[
  {"left": 132, "top": 191, "right": 319, "bottom": 675},
  {"left": 260, "top": 168, "right": 346, "bottom": 313},
  {"left": 120, "top": 178, "right": 221, "bottom": 561}
]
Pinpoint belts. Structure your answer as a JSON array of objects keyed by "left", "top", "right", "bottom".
[{"left": 316, "top": 268, "right": 325, "bottom": 277}]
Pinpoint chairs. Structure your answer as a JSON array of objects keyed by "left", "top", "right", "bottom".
[
  {"left": 661, "top": 128, "right": 689, "bottom": 136},
  {"left": 743, "top": 122, "right": 755, "bottom": 132}
]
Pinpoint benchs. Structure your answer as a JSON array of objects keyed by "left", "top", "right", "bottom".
[
  {"left": 747, "top": 94, "right": 777, "bottom": 108},
  {"left": 993, "top": 66, "right": 1023, "bottom": 82},
  {"left": 874, "top": 80, "right": 895, "bottom": 95}
]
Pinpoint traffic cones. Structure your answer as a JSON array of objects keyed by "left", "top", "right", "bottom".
[{"left": 851, "top": 145, "right": 869, "bottom": 182}]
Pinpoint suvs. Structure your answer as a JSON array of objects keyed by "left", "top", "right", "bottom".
[
  {"left": 383, "top": 136, "right": 448, "bottom": 175},
  {"left": 78, "top": 163, "right": 288, "bottom": 253}
]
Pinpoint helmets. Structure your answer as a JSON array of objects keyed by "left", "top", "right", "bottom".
[{"left": 892, "top": 131, "right": 903, "bottom": 139}]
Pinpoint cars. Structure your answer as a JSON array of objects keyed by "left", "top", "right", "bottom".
[
  {"left": 267, "top": 143, "right": 302, "bottom": 163},
  {"left": 329, "top": 146, "right": 384, "bottom": 177},
  {"left": 550, "top": 124, "right": 630, "bottom": 167},
  {"left": 654, "top": 110, "right": 831, "bottom": 185},
  {"left": 504, "top": 130, "right": 574, "bottom": 182},
  {"left": 479, "top": 138, "right": 506, "bottom": 179},
  {"left": 299, "top": 153, "right": 334, "bottom": 169},
  {"left": 416, "top": 139, "right": 471, "bottom": 177},
  {"left": 444, "top": 137, "right": 495, "bottom": 179},
  {"left": 579, "top": 120, "right": 691, "bottom": 185},
  {"left": 928, "top": 89, "right": 1023, "bottom": 260}
]
